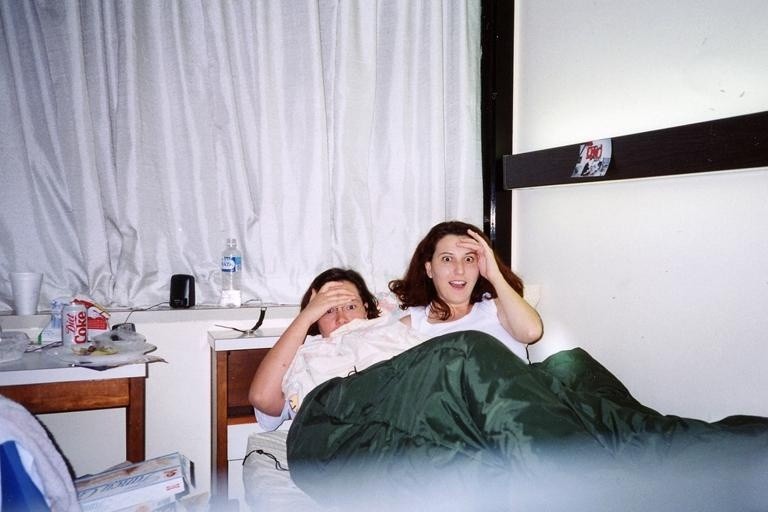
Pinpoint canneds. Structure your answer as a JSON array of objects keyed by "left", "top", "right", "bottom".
[{"left": 62, "top": 302, "right": 88, "bottom": 347}]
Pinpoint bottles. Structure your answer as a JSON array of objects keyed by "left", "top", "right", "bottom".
[{"left": 216, "top": 237, "right": 244, "bottom": 307}]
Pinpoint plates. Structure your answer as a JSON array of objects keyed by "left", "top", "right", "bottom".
[{"left": 45, "top": 341, "right": 158, "bottom": 364}]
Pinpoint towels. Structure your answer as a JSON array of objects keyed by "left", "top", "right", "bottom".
[{"left": 0, "top": 394, "right": 82, "bottom": 511}]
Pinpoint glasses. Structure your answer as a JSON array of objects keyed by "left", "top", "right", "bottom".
[{"left": 325, "top": 302, "right": 365, "bottom": 317}]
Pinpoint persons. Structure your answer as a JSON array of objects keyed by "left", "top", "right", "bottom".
[
  {"left": 247, "top": 267, "right": 421, "bottom": 434},
  {"left": 389, "top": 221, "right": 544, "bottom": 367}
]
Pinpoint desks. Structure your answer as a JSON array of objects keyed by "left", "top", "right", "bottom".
[{"left": 0, "top": 339, "right": 151, "bottom": 466}]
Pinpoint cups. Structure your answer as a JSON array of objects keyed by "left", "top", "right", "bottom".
[{"left": 6, "top": 270, "right": 45, "bottom": 316}]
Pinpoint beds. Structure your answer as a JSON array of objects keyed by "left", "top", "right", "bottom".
[{"left": 205, "top": 322, "right": 767, "bottom": 511}]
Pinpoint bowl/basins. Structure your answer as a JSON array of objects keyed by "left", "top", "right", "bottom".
[{"left": 1, "top": 331, "right": 31, "bottom": 364}]
